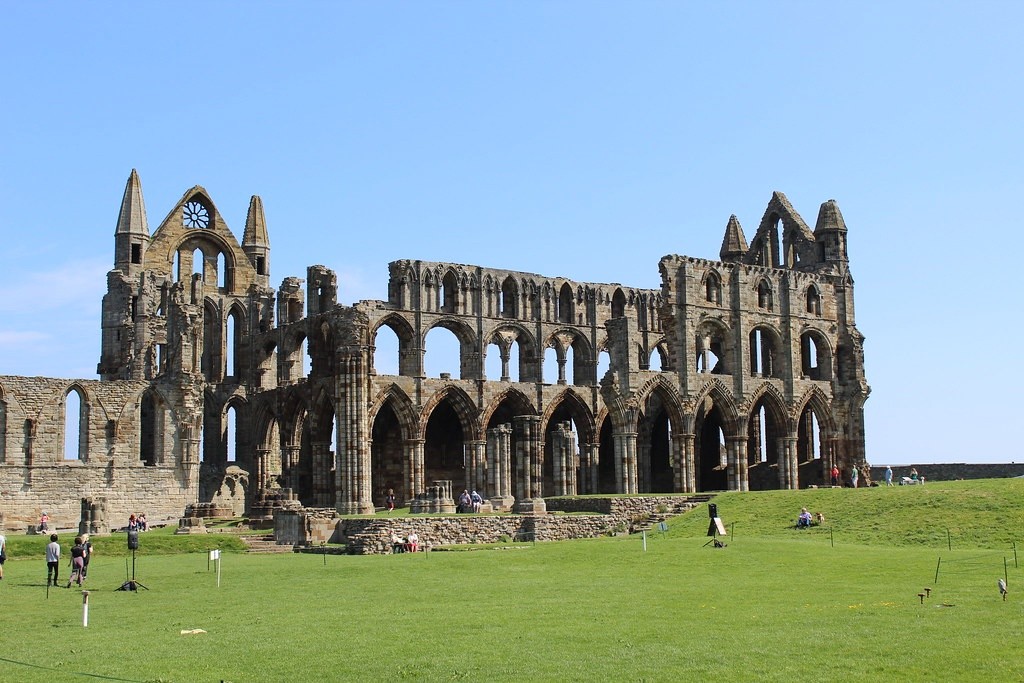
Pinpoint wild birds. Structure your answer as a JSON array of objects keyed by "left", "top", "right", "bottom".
[{"left": 997, "top": 578, "right": 1008, "bottom": 594}]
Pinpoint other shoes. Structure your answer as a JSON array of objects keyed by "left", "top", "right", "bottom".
[
  {"left": 68, "top": 582, "right": 71, "bottom": 588},
  {"left": 79, "top": 584, "right": 82, "bottom": 587}
]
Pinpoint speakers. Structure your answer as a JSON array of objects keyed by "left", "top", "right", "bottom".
[
  {"left": 128, "top": 531, "right": 139, "bottom": 549},
  {"left": 708, "top": 504, "right": 717, "bottom": 517}
]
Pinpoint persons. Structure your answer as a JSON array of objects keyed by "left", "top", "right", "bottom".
[
  {"left": 389, "top": 528, "right": 419, "bottom": 554},
  {"left": 885, "top": 465, "right": 894, "bottom": 486},
  {"left": 458, "top": 490, "right": 472, "bottom": 513},
  {"left": 387, "top": 488, "right": 395, "bottom": 516},
  {"left": 46, "top": 534, "right": 60, "bottom": 587},
  {"left": 831, "top": 464, "right": 839, "bottom": 487},
  {"left": 909, "top": 467, "right": 918, "bottom": 480},
  {"left": 67, "top": 538, "right": 86, "bottom": 588},
  {"left": 471, "top": 491, "right": 483, "bottom": 514},
  {"left": 851, "top": 465, "right": 859, "bottom": 489},
  {"left": 0, "top": 535, "right": 6, "bottom": 580},
  {"left": 40, "top": 512, "right": 50, "bottom": 531},
  {"left": 76, "top": 533, "right": 93, "bottom": 580},
  {"left": 796, "top": 508, "right": 813, "bottom": 528},
  {"left": 129, "top": 511, "right": 153, "bottom": 533}
]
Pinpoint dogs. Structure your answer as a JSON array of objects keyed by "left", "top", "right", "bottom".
[
  {"left": 815, "top": 512, "right": 825, "bottom": 526},
  {"left": 844, "top": 481, "right": 854, "bottom": 488}
]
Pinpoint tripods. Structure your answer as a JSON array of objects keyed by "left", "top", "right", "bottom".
[
  {"left": 703, "top": 517, "right": 723, "bottom": 548},
  {"left": 114, "top": 549, "right": 148, "bottom": 594}
]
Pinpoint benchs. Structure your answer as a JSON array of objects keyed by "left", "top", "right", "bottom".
[{"left": 391, "top": 543, "right": 431, "bottom": 552}]
